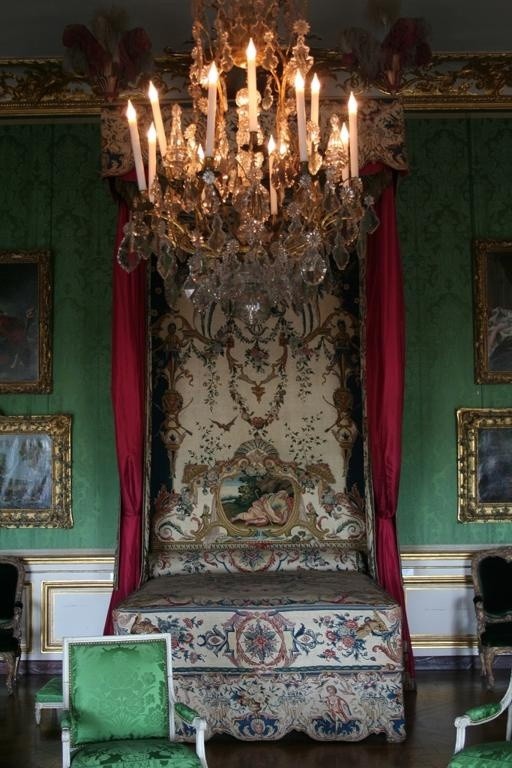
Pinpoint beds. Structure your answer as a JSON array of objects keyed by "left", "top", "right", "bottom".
[{"left": 109, "top": 438, "right": 408, "bottom": 745}]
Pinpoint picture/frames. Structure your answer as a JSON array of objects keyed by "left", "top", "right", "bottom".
[
  {"left": 0, "top": 415, "right": 74, "bottom": 528},
  {"left": 0, "top": 250, "right": 54, "bottom": 394},
  {"left": 456, "top": 407, "right": 512, "bottom": 523},
  {"left": 470, "top": 239, "right": 512, "bottom": 385}
]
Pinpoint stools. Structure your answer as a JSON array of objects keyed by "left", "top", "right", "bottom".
[{"left": 34, "top": 677, "right": 63, "bottom": 726}]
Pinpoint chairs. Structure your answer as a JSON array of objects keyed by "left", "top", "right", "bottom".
[
  {"left": 471, "top": 546, "right": 512, "bottom": 689},
  {"left": 60, "top": 633, "right": 209, "bottom": 768},
  {"left": 445, "top": 668, "right": 512, "bottom": 768},
  {"left": 0, "top": 554, "right": 26, "bottom": 698}
]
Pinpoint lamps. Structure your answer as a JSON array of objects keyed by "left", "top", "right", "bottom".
[{"left": 116, "top": 0, "right": 383, "bottom": 326}]
